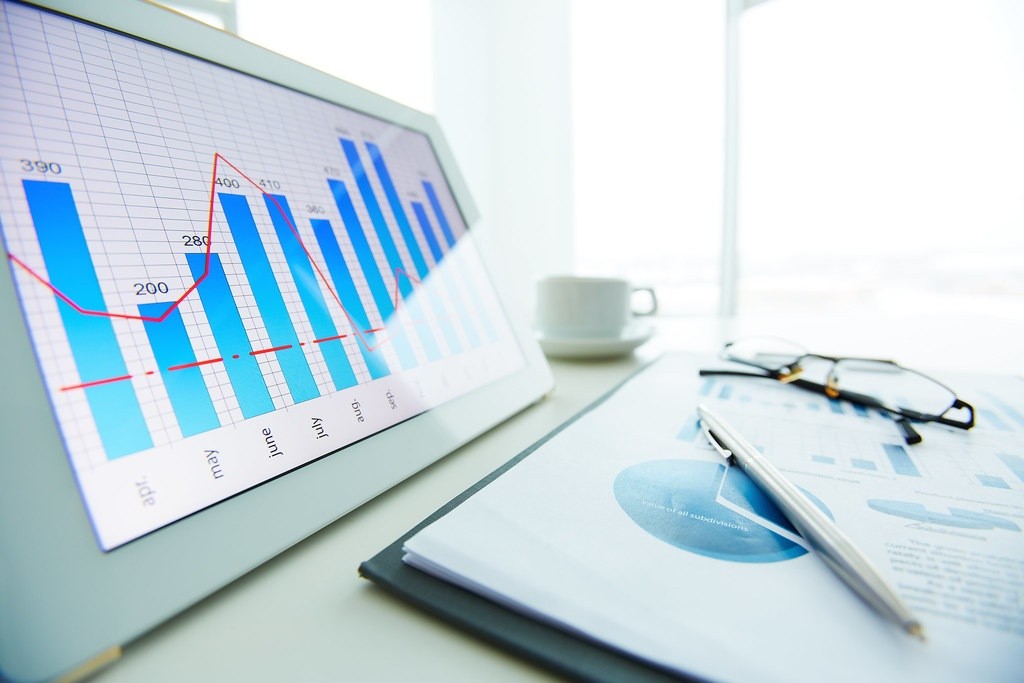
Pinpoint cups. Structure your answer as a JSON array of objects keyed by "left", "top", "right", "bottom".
[{"left": 536, "top": 276, "right": 657, "bottom": 341}]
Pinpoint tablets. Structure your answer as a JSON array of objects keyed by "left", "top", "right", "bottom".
[{"left": 0, "top": 0, "right": 549, "bottom": 683}]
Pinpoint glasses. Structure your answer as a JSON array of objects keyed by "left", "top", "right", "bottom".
[{"left": 701, "top": 332, "right": 974, "bottom": 444}]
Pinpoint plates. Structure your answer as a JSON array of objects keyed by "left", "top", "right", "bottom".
[{"left": 533, "top": 337, "right": 653, "bottom": 357}]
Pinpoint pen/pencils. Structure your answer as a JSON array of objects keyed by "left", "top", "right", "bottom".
[{"left": 690, "top": 400, "right": 933, "bottom": 644}]
